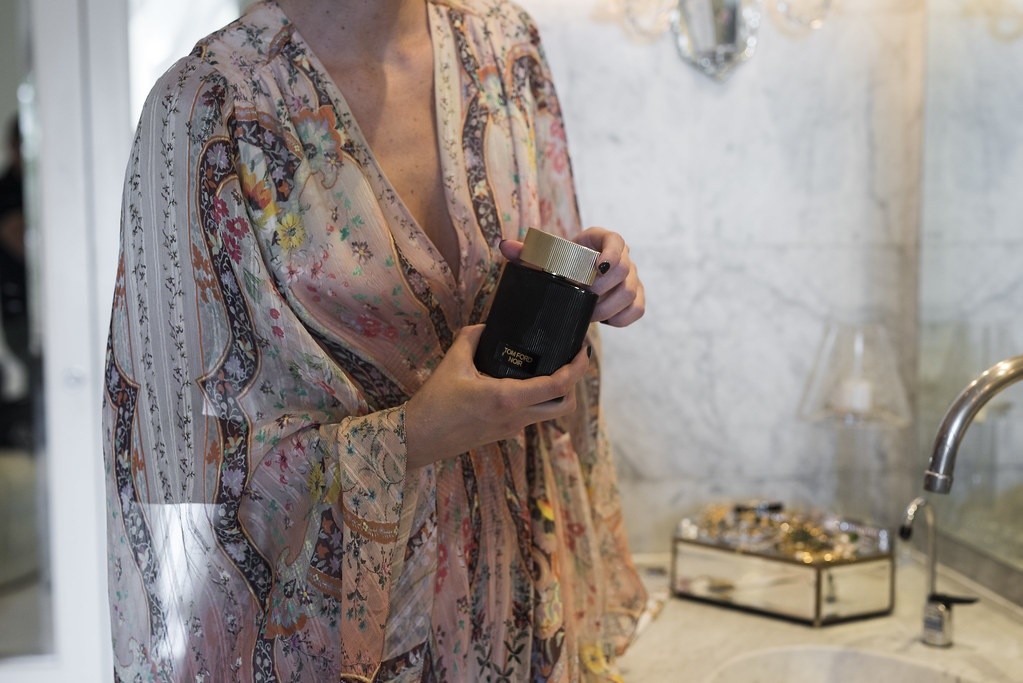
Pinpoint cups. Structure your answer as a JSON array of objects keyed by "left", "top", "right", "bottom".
[{"left": 473, "top": 226, "right": 601, "bottom": 403}]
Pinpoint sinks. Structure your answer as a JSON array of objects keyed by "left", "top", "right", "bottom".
[{"left": 705, "top": 643, "right": 977, "bottom": 683}]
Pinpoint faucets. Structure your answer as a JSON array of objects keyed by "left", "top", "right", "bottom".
[
  {"left": 921, "top": 355, "right": 1023, "bottom": 495},
  {"left": 898, "top": 497, "right": 980, "bottom": 649}
]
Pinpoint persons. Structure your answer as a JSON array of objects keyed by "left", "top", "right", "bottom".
[
  {"left": 101, "top": 0, "right": 647, "bottom": 683},
  {"left": 0, "top": 116, "right": 33, "bottom": 453}
]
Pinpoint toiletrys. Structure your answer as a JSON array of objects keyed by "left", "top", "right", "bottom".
[{"left": 471, "top": 226, "right": 602, "bottom": 381}]
